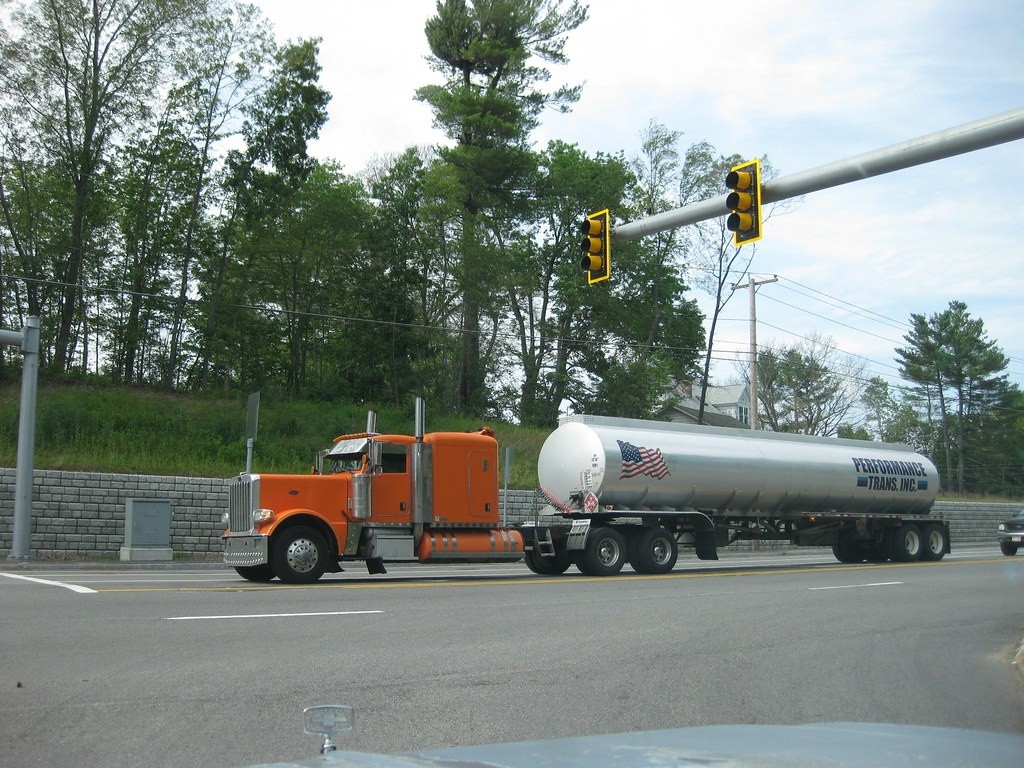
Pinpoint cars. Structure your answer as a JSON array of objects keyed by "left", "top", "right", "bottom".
[{"left": 997, "top": 507, "right": 1024, "bottom": 557}]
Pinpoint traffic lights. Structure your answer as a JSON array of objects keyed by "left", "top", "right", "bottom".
[
  {"left": 581, "top": 209, "right": 610, "bottom": 285},
  {"left": 725, "top": 158, "right": 762, "bottom": 247}
]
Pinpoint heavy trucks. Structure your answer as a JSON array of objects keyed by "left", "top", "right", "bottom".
[{"left": 220, "top": 396, "right": 952, "bottom": 585}]
